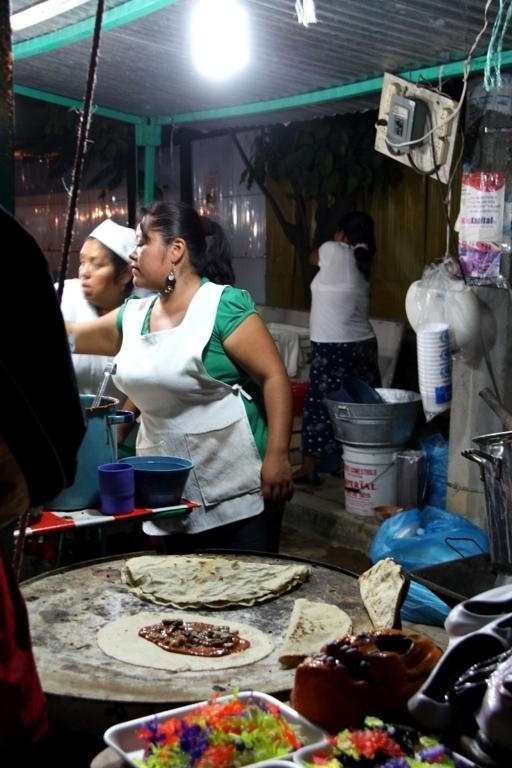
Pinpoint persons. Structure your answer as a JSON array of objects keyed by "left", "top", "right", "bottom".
[
  {"left": 0, "top": 203, "right": 88, "bottom": 684},
  {"left": 49, "top": 218, "right": 142, "bottom": 447},
  {"left": 63, "top": 198, "right": 294, "bottom": 554}
]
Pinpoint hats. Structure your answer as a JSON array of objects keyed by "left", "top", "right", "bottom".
[{"left": 90, "top": 218, "right": 136, "bottom": 264}]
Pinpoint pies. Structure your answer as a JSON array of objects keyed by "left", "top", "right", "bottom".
[
  {"left": 356, "top": 553, "right": 407, "bottom": 630},
  {"left": 93, "top": 609, "right": 274, "bottom": 673},
  {"left": 121, "top": 553, "right": 313, "bottom": 605},
  {"left": 278, "top": 598, "right": 352, "bottom": 666}
]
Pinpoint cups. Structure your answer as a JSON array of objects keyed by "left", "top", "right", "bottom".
[
  {"left": 416, "top": 324, "right": 452, "bottom": 417},
  {"left": 97, "top": 462, "right": 134, "bottom": 514}
]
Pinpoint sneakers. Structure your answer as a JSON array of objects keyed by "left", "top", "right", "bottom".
[{"left": 292, "top": 468, "right": 320, "bottom": 486}]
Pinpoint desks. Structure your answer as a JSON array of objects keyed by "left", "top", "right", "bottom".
[{"left": 12, "top": 496, "right": 201, "bottom": 569}]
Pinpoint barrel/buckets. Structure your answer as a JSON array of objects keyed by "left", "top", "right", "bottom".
[{"left": 337, "top": 443, "right": 397, "bottom": 516}]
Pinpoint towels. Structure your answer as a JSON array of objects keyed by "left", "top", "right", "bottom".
[{"left": 274, "top": 330, "right": 305, "bottom": 377}]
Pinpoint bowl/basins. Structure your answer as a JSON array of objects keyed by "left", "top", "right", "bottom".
[
  {"left": 324, "top": 387, "right": 423, "bottom": 450},
  {"left": 116, "top": 452, "right": 193, "bottom": 505}
]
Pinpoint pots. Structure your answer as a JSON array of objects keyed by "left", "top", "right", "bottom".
[{"left": 460, "top": 424, "right": 512, "bottom": 570}]
